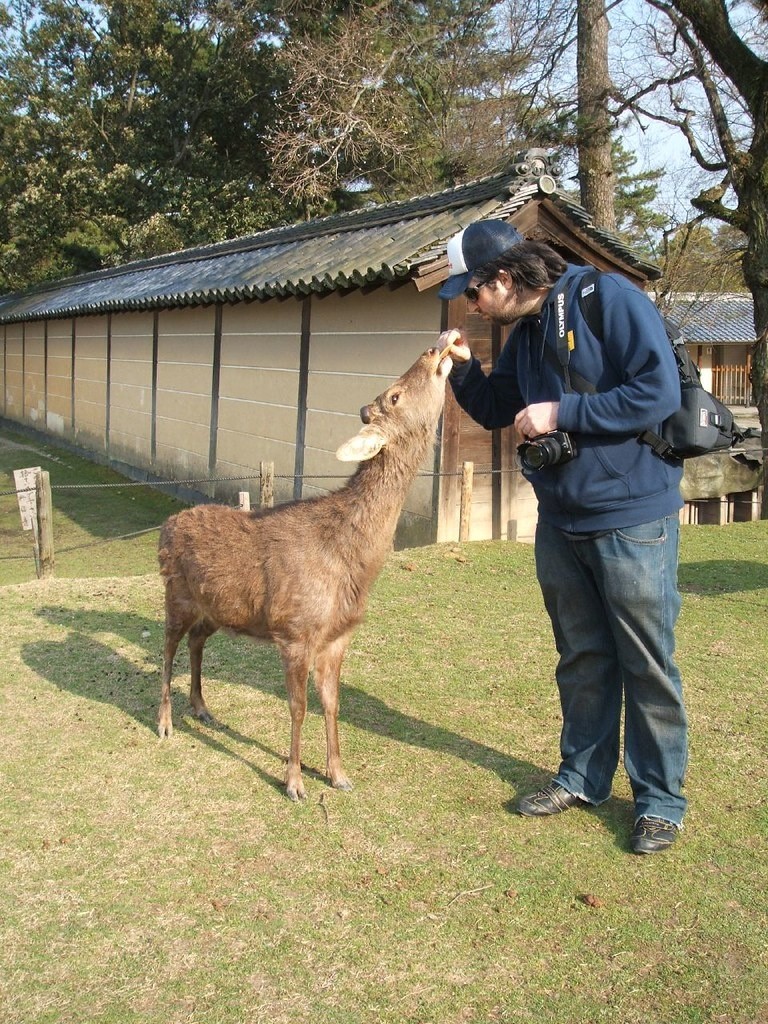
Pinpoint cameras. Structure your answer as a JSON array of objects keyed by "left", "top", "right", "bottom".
[{"left": 513, "top": 430, "right": 579, "bottom": 475}]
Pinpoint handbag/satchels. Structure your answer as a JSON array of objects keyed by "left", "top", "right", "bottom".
[{"left": 578, "top": 271, "right": 744, "bottom": 467}]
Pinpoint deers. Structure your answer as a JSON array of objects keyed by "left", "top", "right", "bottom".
[{"left": 155, "top": 344, "right": 457, "bottom": 800}]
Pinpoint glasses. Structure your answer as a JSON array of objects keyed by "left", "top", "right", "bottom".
[{"left": 463, "top": 278, "right": 490, "bottom": 301}]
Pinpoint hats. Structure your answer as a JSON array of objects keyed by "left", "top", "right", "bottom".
[{"left": 438, "top": 218, "right": 523, "bottom": 299}]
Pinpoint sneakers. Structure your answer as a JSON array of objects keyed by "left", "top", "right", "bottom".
[
  {"left": 632, "top": 815, "right": 677, "bottom": 853},
  {"left": 516, "top": 779, "right": 584, "bottom": 816}
]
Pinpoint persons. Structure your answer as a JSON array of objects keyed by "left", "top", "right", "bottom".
[{"left": 439, "top": 220, "right": 690, "bottom": 857}]
